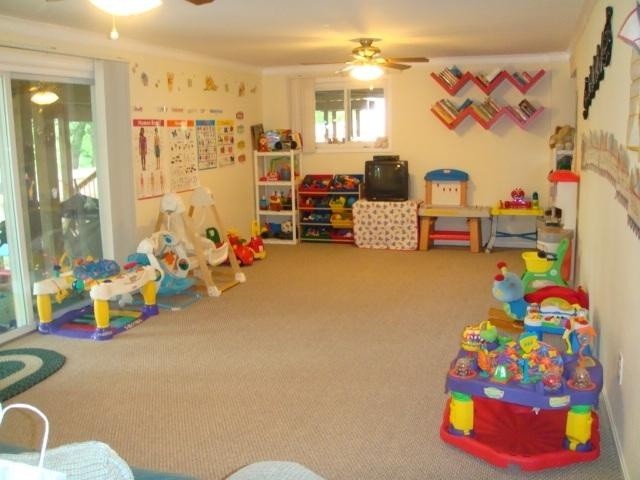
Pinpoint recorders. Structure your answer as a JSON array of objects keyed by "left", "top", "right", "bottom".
[{"left": 275, "top": 141, "right": 297, "bottom": 150}]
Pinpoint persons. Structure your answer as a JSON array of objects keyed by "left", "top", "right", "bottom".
[
  {"left": 153, "top": 127, "right": 161, "bottom": 169},
  {"left": 138, "top": 126, "right": 148, "bottom": 169}
]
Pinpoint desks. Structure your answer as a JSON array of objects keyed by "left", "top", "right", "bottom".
[{"left": 485, "top": 205, "right": 544, "bottom": 253}]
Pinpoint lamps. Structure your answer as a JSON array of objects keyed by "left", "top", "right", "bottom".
[
  {"left": 86, "top": 0, "right": 164, "bottom": 40},
  {"left": 28, "top": 81, "right": 59, "bottom": 105},
  {"left": 349, "top": 63, "right": 384, "bottom": 81}
]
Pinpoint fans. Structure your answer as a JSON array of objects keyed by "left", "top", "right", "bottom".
[
  {"left": 299, "top": 38, "right": 429, "bottom": 71},
  {"left": 47, "top": 0, "right": 214, "bottom": 5}
]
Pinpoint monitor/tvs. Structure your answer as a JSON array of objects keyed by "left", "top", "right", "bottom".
[{"left": 365, "top": 160, "right": 408, "bottom": 200}]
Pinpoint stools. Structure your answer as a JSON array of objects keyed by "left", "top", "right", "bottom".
[{"left": 418, "top": 206, "right": 491, "bottom": 253}]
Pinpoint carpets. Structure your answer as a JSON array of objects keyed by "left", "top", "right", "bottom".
[{"left": 0, "top": 348, "right": 66, "bottom": 403}]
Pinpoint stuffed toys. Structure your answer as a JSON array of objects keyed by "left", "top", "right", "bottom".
[
  {"left": 546, "top": 124, "right": 575, "bottom": 150},
  {"left": 491, "top": 261, "right": 530, "bottom": 321}
]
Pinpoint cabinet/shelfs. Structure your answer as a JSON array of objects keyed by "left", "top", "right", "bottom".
[
  {"left": 296, "top": 183, "right": 362, "bottom": 243},
  {"left": 254, "top": 148, "right": 301, "bottom": 245},
  {"left": 430, "top": 68, "right": 546, "bottom": 131}
]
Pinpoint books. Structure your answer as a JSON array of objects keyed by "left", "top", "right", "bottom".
[{"left": 432, "top": 61, "right": 538, "bottom": 125}]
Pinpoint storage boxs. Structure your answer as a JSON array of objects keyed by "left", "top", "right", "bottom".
[
  {"left": 304, "top": 211, "right": 331, "bottom": 223},
  {"left": 301, "top": 227, "right": 331, "bottom": 240},
  {"left": 314, "top": 201, "right": 329, "bottom": 214},
  {"left": 329, "top": 214, "right": 353, "bottom": 228},
  {"left": 330, "top": 230, "right": 354, "bottom": 243},
  {"left": 299, "top": 198, "right": 316, "bottom": 211},
  {"left": 330, "top": 174, "right": 363, "bottom": 197},
  {"left": 298, "top": 175, "right": 333, "bottom": 196},
  {"left": 329, "top": 198, "right": 344, "bottom": 213}
]
